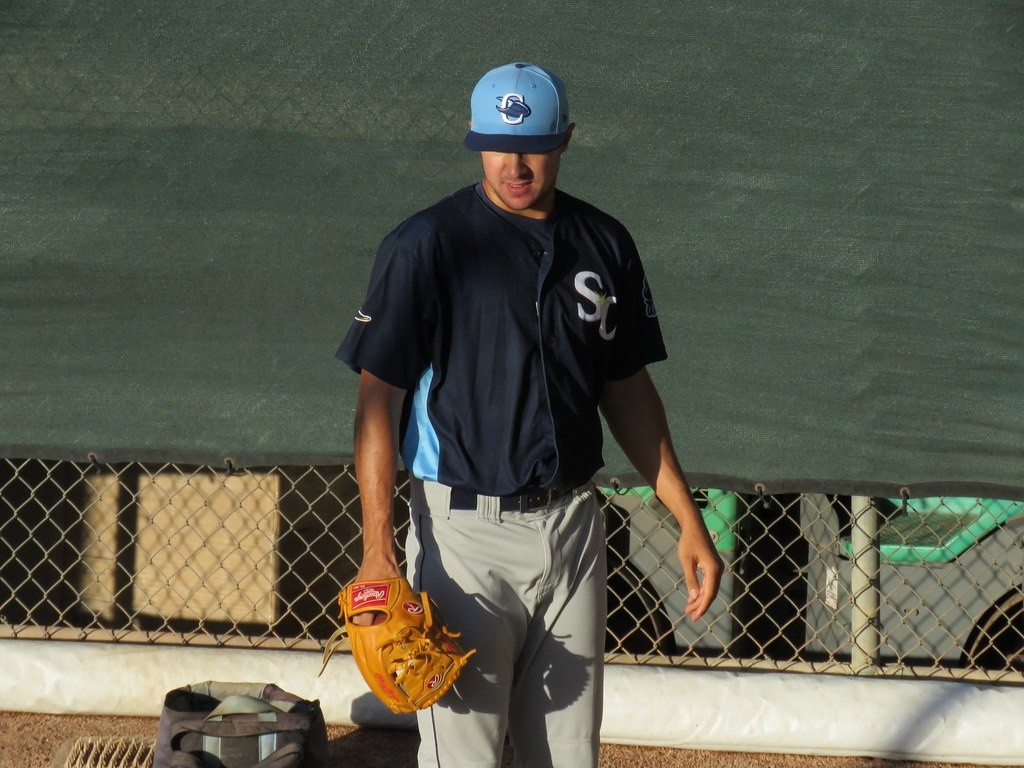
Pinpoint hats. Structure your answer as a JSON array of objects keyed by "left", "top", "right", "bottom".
[{"left": 463, "top": 60, "right": 571, "bottom": 154}]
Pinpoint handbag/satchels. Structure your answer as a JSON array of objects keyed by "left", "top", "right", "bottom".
[{"left": 154, "top": 680, "right": 327, "bottom": 768}]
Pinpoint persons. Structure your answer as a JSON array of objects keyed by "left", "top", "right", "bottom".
[{"left": 334, "top": 60, "right": 725, "bottom": 768}]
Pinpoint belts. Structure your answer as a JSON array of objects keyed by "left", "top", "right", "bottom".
[{"left": 449, "top": 486, "right": 572, "bottom": 511}]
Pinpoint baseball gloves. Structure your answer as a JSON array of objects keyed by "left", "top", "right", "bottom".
[{"left": 336, "top": 577, "right": 479, "bottom": 715}]
